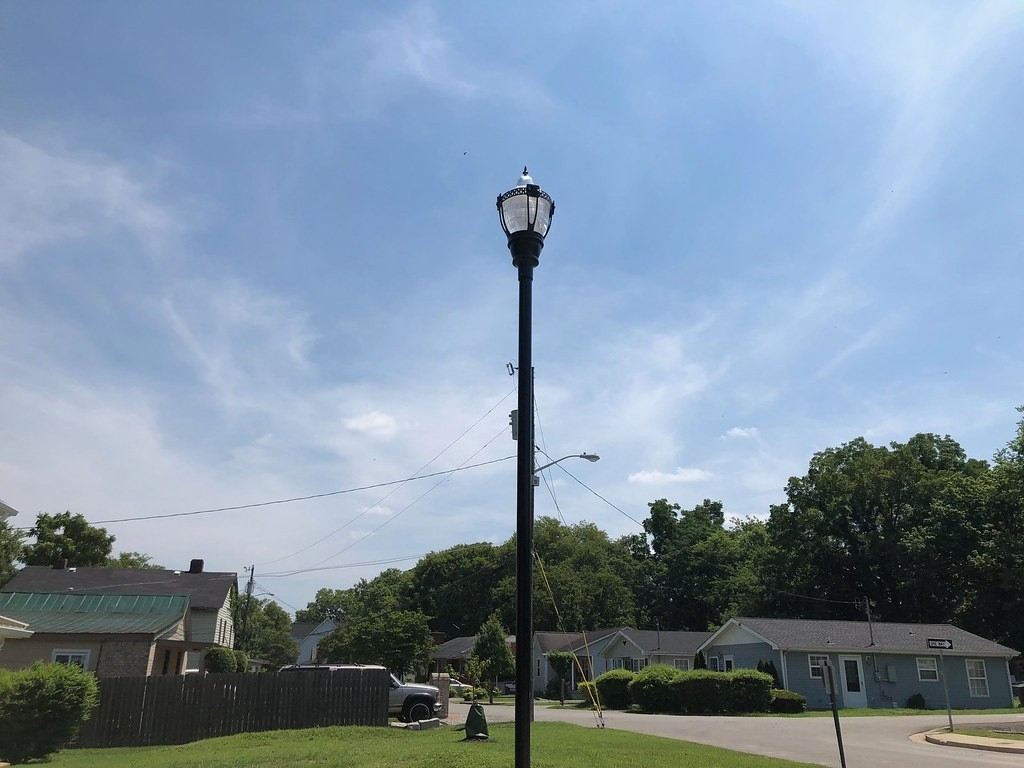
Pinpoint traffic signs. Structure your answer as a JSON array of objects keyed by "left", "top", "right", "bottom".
[{"left": 927, "top": 639, "right": 953, "bottom": 649}]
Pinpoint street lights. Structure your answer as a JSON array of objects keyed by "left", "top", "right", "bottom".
[
  {"left": 531, "top": 452, "right": 600, "bottom": 722},
  {"left": 242, "top": 592, "right": 274, "bottom": 638},
  {"left": 497, "top": 164, "right": 557, "bottom": 768}
]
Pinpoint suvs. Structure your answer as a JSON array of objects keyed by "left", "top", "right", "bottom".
[{"left": 277, "top": 664, "right": 443, "bottom": 723}]
